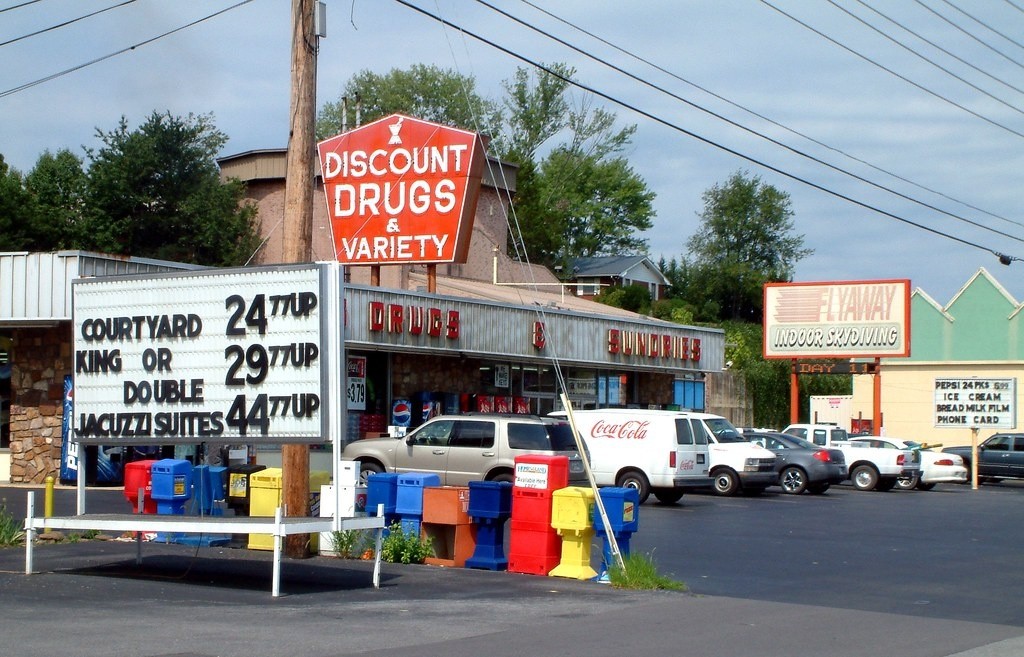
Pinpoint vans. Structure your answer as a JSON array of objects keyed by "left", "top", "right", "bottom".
[
  {"left": 781, "top": 424, "right": 847, "bottom": 449},
  {"left": 691, "top": 412, "right": 779, "bottom": 497},
  {"left": 546, "top": 409, "right": 716, "bottom": 505}
]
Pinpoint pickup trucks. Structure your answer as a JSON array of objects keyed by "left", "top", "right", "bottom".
[{"left": 780, "top": 423, "right": 920, "bottom": 491}]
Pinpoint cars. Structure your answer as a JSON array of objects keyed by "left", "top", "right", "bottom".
[
  {"left": 714, "top": 428, "right": 778, "bottom": 433},
  {"left": 848, "top": 436, "right": 968, "bottom": 490},
  {"left": 740, "top": 432, "right": 847, "bottom": 495}
]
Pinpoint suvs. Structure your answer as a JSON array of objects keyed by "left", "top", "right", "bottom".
[
  {"left": 341, "top": 413, "right": 595, "bottom": 489},
  {"left": 941, "top": 432, "right": 1024, "bottom": 485}
]
